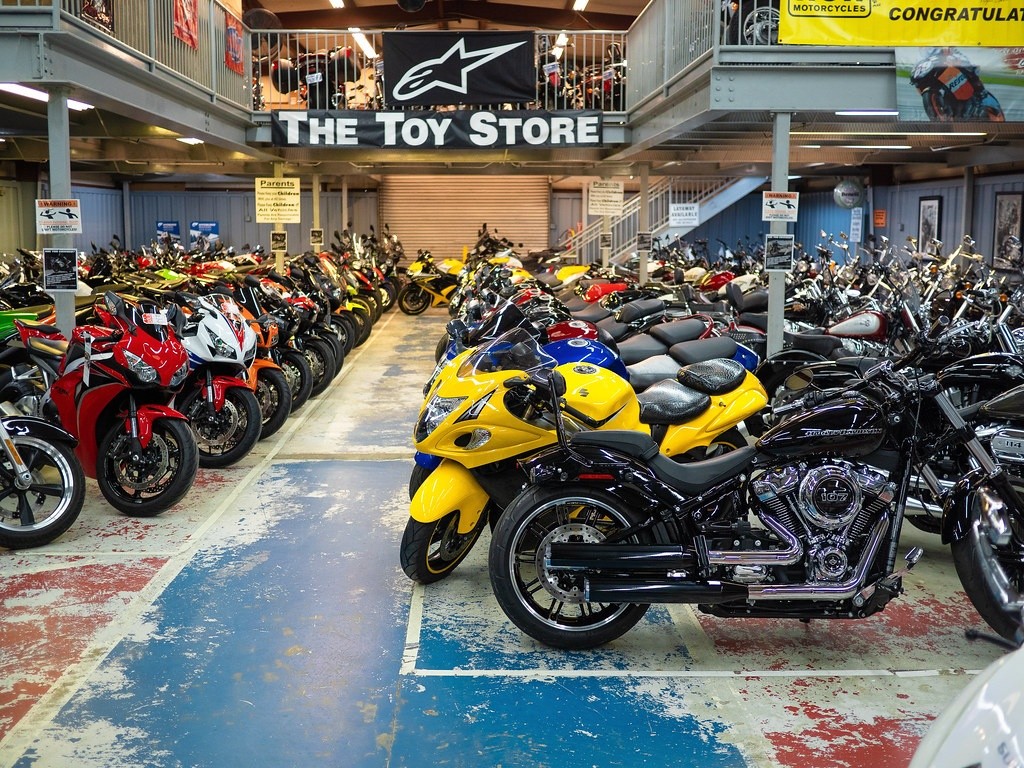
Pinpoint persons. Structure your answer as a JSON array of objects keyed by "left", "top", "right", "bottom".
[
  {"left": 768, "top": 240, "right": 783, "bottom": 255},
  {"left": 922, "top": 219, "right": 933, "bottom": 255}
]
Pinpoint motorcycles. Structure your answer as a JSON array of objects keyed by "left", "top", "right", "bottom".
[
  {"left": 910, "top": 47, "right": 1005, "bottom": 121},
  {"left": 0, "top": 220, "right": 407, "bottom": 551},
  {"left": 242, "top": 55, "right": 267, "bottom": 111},
  {"left": 399, "top": 224, "right": 1024, "bottom": 647},
  {"left": 271, "top": 23, "right": 629, "bottom": 110},
  {"left": 52, "top": 255, "right": 73, "bottom": 273}
]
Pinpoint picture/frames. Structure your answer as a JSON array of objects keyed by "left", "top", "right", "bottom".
[
  {"left": 917, "top": 195, "right": 942, "bottom": 261},
  {"left": 992, "top": 191, "right": 1024, "bottom": 274}
]
